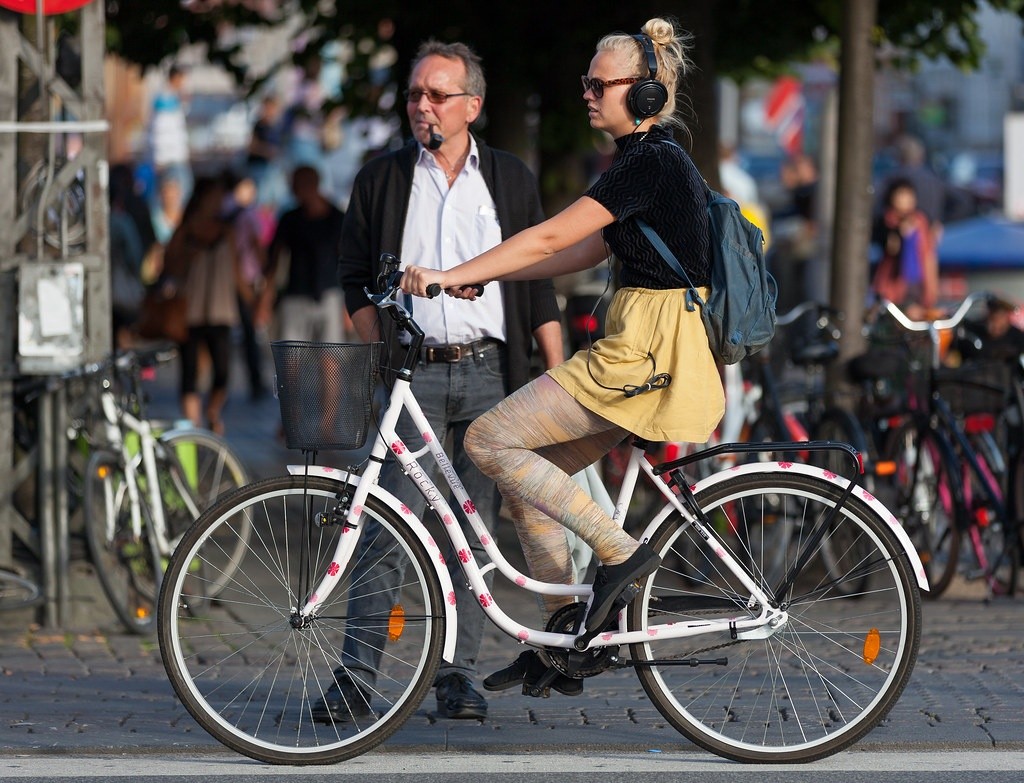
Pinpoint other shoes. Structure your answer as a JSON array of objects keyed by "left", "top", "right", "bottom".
[
  {"left": 482, "top": 649, "right": 584, "bottom": 696},
  {"left": 584, "top": 536, "right": 661, "bottom": 631}
]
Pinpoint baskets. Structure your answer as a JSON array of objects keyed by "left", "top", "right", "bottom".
[{"left": 269, "top": 340, "right": 381, "bottom": 449}]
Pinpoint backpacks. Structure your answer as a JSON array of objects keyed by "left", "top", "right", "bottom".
[{"left": 634, "top": 138, "right": 778, "bottom": 365}]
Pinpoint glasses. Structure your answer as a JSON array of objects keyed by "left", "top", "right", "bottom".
[
  {"left": 404, "top": 89, "right": 468, "bottom": 103},
  {"left": 582, "top": 75, "right": 643, "bottom": 98}
]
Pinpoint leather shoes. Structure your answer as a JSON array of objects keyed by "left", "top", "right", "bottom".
[
  {"left": 435, "top": 673, "right": 488, "bottom": 719},
  {"left": 311, "top": 674, "right": 372, "bottom": 722}
]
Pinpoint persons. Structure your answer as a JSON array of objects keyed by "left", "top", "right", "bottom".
[
  {"left": 311, "top": 42, "right": 566, "bottom": 718},
  {"left": 754, "top": 129, "right": 956, "bottom": 316},
  {"left": 400, "top": 18, "right": 723, "bottom": 692},
  {"left": 103, "top": 62, "right": 357, "bottom": 452}
]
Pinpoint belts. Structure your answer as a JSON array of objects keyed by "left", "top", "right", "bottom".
[{"left": 419, "top": 337, "right": 498, "bottom": 363}]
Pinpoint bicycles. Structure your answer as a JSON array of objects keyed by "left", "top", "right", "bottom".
[
  {"left": 56, "top": 340, "right": 255, "bottom": 638},
  {"left": 157, "top": 251, "right": 936, "bottom": 766},
  {"left": 571, "top": 294, "right": 1024, "bottom": 606}
]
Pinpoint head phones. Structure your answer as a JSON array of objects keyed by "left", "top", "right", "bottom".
[{"left": 626, "top": 34, "right": 668, "bottom": 118}]
